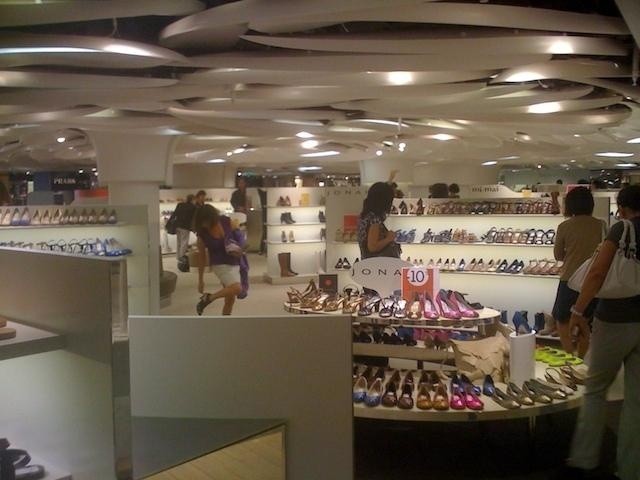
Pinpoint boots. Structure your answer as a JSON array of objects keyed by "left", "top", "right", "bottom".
[
  {"left": 314, "top": 250, "right": 325, "bottom": 275},
  {"left": 279, "top": 253, "right": 298, "bottom": 277}
]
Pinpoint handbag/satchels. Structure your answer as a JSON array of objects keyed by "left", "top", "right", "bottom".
[
  {"left": 567, "top": 250, "right": 640, "bottom": 299},
  {"left": 225, "top": 238, "right": 242, "bottom": 257},
  {"left": 166, "top": 217, "right": 176, "bottom": 234}
]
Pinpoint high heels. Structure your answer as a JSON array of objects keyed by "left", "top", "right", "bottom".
[
  {"left": 197, "top": 293, "right": 211, "bottom": 315},
  {"left": 320, "top": 229, "right": 326, "bottom": 240}
]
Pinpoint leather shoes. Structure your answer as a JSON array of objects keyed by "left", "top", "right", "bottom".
[
  {"left": 280, "top": 196, "right": 296, "bottom": 242},
  {"left": 335, "top": 192, "right": 564, "bottom": 275},
  {"left": 0, "top": 208, "right": 117, "bottom": 225},
  {"left": 287, "top": 280, "right": 586, "bottom": 410},
  {"left": 319, "top": 211, "right": 325, "bottom": 223}
]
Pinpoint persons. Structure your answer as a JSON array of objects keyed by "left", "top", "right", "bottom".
[
  {"left": 551, "top": 186, "right": 609, "bottom": 360},
  {"left": 356, "top": 183, "right": 401, "bottom": 259},
  {"left": 615, "top": 183, "right": 630, "bottom": 219},
  {"left": 556, "top": 179, "right": 562, "bottom": 184},
  {"left": 567, "top": 183, "right": 640, "bottom": 480},
  {"left": 176, "top": 179, "right": 249, "bottom": 315}
]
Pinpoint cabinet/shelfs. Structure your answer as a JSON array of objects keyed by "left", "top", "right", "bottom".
[
  {"left": 282, "top": 256, "right": 589, "bottom": 423},
  {"left": 0, "top": 204, "right": 152, "bottom": 316},
  {"left": 158, "top": 186, "right": 240, "bottom": 255},
  {"left": 262, "top": 185, "right": 325, "bottom": 286}
]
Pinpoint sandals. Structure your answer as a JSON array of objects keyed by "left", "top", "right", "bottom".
[{"left": 0, "top": 237, "right": 132, "bottom": 256}]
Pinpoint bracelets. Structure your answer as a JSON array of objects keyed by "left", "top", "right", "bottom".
[{"left": 570, "top": 304, "right": 583, "bottom": 316}]
